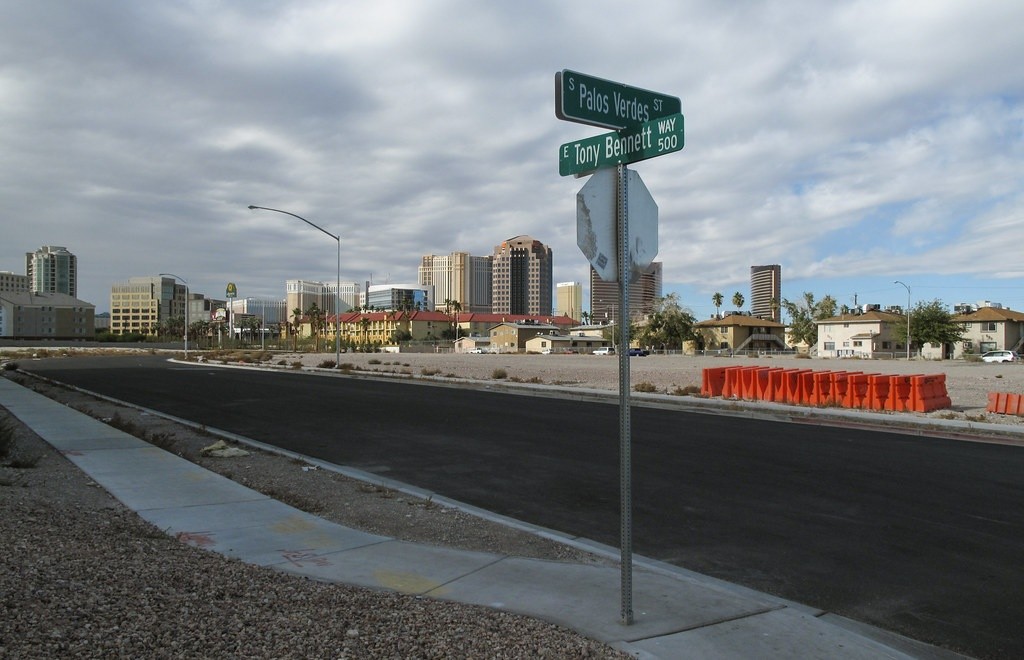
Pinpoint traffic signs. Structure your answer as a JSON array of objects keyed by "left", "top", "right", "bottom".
[{"left": 552, "top": 68, "right": 685, "bottom": 180}]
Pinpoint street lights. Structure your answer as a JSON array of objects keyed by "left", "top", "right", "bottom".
[
  {"left": 894, "top": 280, "right": 911, "bottom": 360},
  {"left": 247, "top": 204, "right": 341, "bottom": 370},
  {"left": 600, "top": 299, "right": 615, "bottom": 347},
  {"left": 158, "top": 272, "right": 188, "bottom": 360}
]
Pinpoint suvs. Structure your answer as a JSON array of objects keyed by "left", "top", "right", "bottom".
[{"left": 980, "top": 350, "right": 1018, "bottom": 362}]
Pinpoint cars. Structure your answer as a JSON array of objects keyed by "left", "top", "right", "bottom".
[
  {"left": 541, "top": 345, "right": 648, "bottom": 356},
  {"left": 470, "top": 347, "right": 482, "bottom": 353}
]
